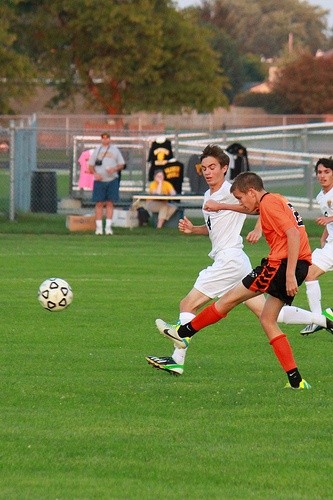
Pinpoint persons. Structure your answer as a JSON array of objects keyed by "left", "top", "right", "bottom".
[
  {"left": 145, "top": 144, "right": 333, "bottom": 391},
  {"left": 88, "top": 130, "right": 125, "bottom": 237},
  {"left": 146, "top": 172, "right": 178, "bottom": 229}
]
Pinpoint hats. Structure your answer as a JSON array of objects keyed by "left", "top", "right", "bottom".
[{"left": 100, "top": 132, "right": 110, "bottom": 136}]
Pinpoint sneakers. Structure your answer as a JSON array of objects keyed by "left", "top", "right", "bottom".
[
  {"left": 155, "top": 319, "right": 190, "bottom": 349},
  {"left": 284, "top": 378, "right": 311, "bottom": 389},
  {"left": 299, "top": 324, "right": 324, "bottom": 336},
  {"left": 321, "top": 307, "right": 333, "bottom": 334},
  {"left": 145, "top": 355, "right": 184, "bottom": 375}
]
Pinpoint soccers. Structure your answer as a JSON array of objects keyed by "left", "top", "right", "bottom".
[{"left": 38, "top": 278, "right": 74, "bottom": 313}]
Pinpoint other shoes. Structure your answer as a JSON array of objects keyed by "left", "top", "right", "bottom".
[
  {"left": 106, "top": 229, "right": 113, "bottom": 235},
  {"left": 95, "top": 229, "right": 102, "bottom": 235}
]
